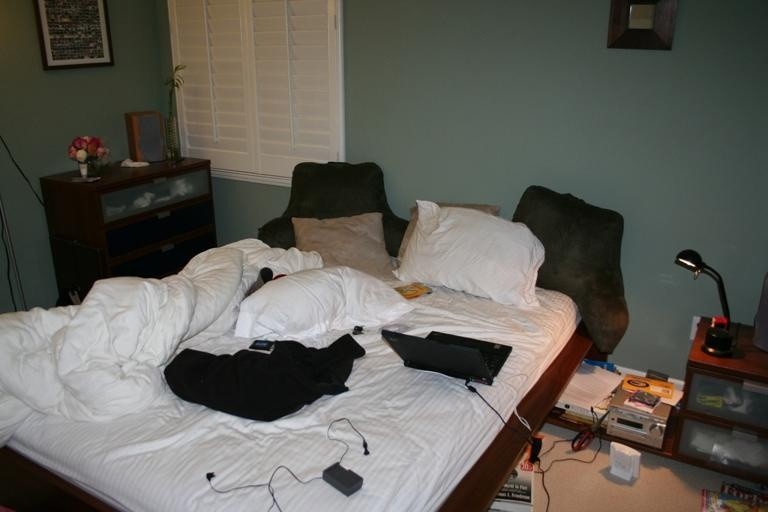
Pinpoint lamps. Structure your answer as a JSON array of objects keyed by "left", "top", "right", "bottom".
[{"left": 673, "top": 245, "right": 738, "bottom": 356}]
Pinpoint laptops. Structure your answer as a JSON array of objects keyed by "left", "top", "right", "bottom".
[{"left": 381, "top": 329, "right": 512, "bottom": 386}]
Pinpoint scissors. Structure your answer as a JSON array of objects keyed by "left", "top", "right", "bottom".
[{"left": 572, "top": 409, "right": 611, "bottom": 452}]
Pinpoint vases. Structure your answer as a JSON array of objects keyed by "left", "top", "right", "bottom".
[{"left": 77, "top": 158, "right": 99, "bottom": 179}]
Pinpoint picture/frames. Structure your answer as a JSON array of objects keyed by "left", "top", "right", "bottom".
[{"left": 33, "top": 0, "right": 116, "bottom": 71}]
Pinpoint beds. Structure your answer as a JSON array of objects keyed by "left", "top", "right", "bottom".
[{"left": 2, "top": 236, "right": 590, "bottom": 512}]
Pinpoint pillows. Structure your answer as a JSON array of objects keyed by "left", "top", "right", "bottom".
[
  {"left": 286, "top": 210, "right": 396, "bottom": 280},
  {"left": 390, "top": 200, "right": 549, "bottom": 314},
  {"left": 398, "top": 203, "right": 500, "bottom": 262}
]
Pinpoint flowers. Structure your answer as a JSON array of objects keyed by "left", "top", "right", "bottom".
[{"left": 66, "top": 134, "right": 110, "bottom": 163}]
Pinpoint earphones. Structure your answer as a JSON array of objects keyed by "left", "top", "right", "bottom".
[
  {"left": 207, "top": 472, "right": 215, "bottom": 479},
  {"left": 363, "top": 448, "right": 370, "bottom": 456}
]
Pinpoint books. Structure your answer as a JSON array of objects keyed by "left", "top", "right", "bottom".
[{"left": 623, "top": 390, "right": 662, "bottom": 413}]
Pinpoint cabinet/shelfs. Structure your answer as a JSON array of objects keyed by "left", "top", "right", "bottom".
[
  {"left": 39, "top": 157, "right": 218, "bottom": 308},
  {"left": 672, "top": 317, "right": 767, "bottom": 490}
]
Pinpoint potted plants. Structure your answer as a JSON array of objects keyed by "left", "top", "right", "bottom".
[{"left": 164, "top": 64, "right": 188, "bottom": 161}]
